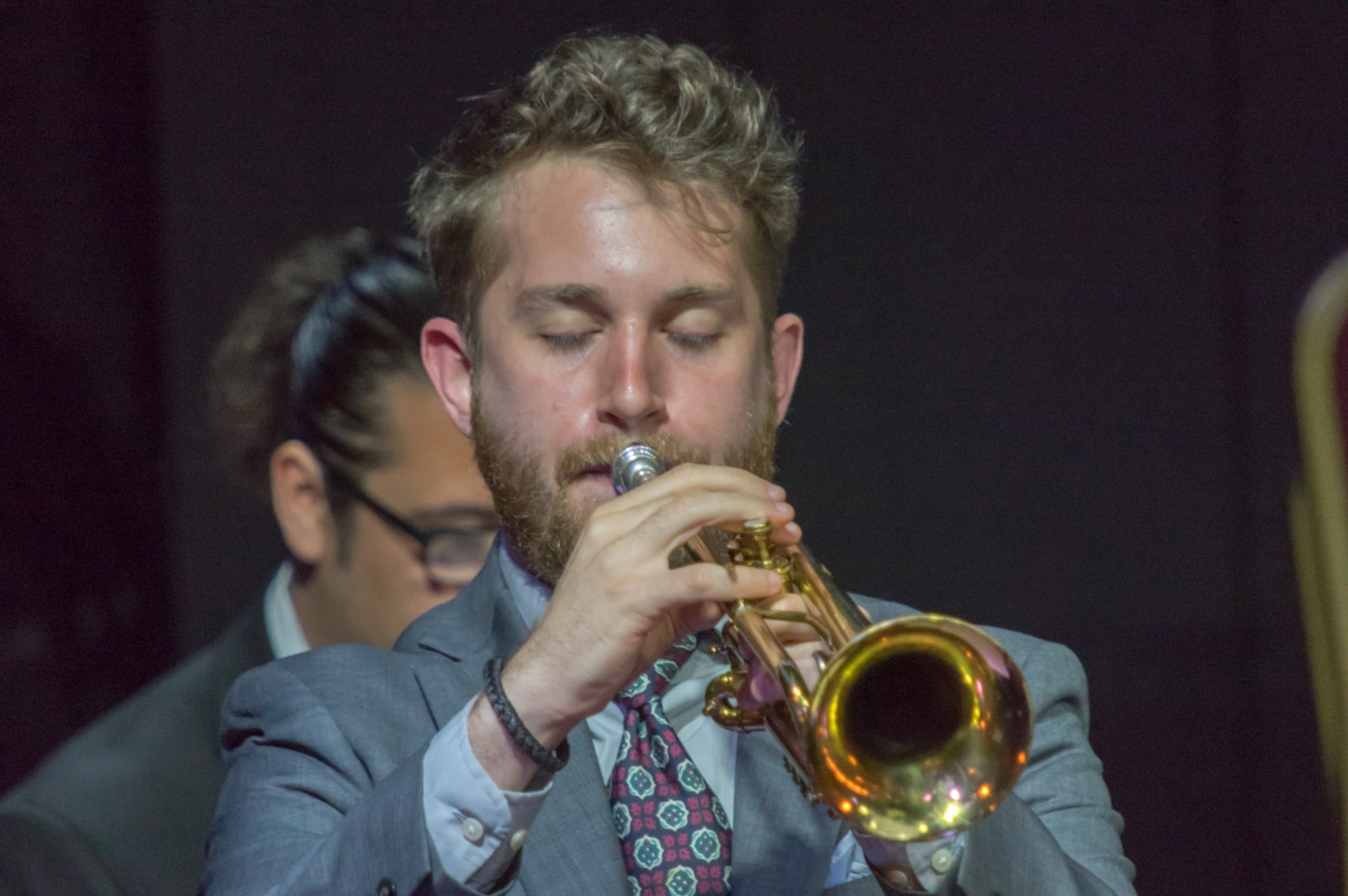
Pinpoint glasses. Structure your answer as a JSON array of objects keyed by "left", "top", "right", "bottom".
[{"left": 318, "top": 460, "right": 501, "bottom": 589}]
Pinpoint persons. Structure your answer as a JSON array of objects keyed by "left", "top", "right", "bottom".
[
  {"left": 203, "top": 33, "right": 1139, "bottom": 896},
  {"left": 0, "top": 231, "right": 498, "bottom": 896}
]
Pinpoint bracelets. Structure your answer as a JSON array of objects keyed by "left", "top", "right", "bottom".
[{"left": 481, "top": 656, "right": 572, "bottom": 771}]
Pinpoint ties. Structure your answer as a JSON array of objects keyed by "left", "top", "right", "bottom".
[{"left": 608, "top": 630, "right": 731, "bottom": 895}]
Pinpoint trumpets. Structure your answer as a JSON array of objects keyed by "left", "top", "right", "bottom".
[{"left": 608, "top": 443, "right": 1033, "bottom": 841}]
